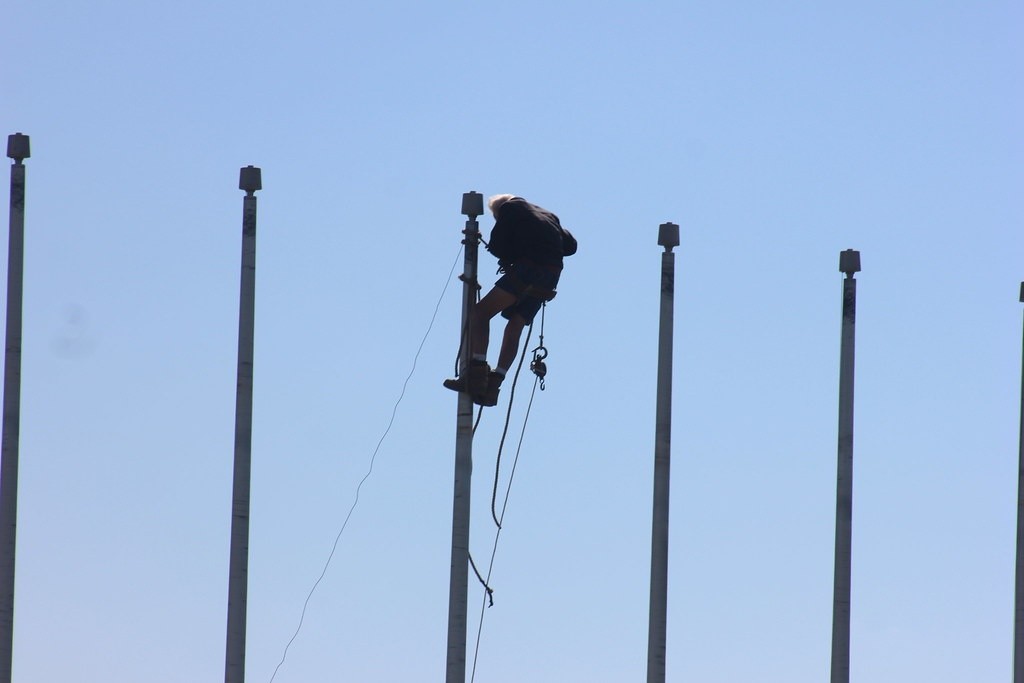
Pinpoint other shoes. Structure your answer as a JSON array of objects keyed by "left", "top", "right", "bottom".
[
  {"left": 442, "top": 360, "right": 492, "bottom": 393},
  {"left": 471, "top": 376, "right": 500, "bottom": 407}
]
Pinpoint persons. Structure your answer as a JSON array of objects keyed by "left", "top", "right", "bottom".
[{"left": 442, "top": 193, "right": 577, "bottom": 409}]
